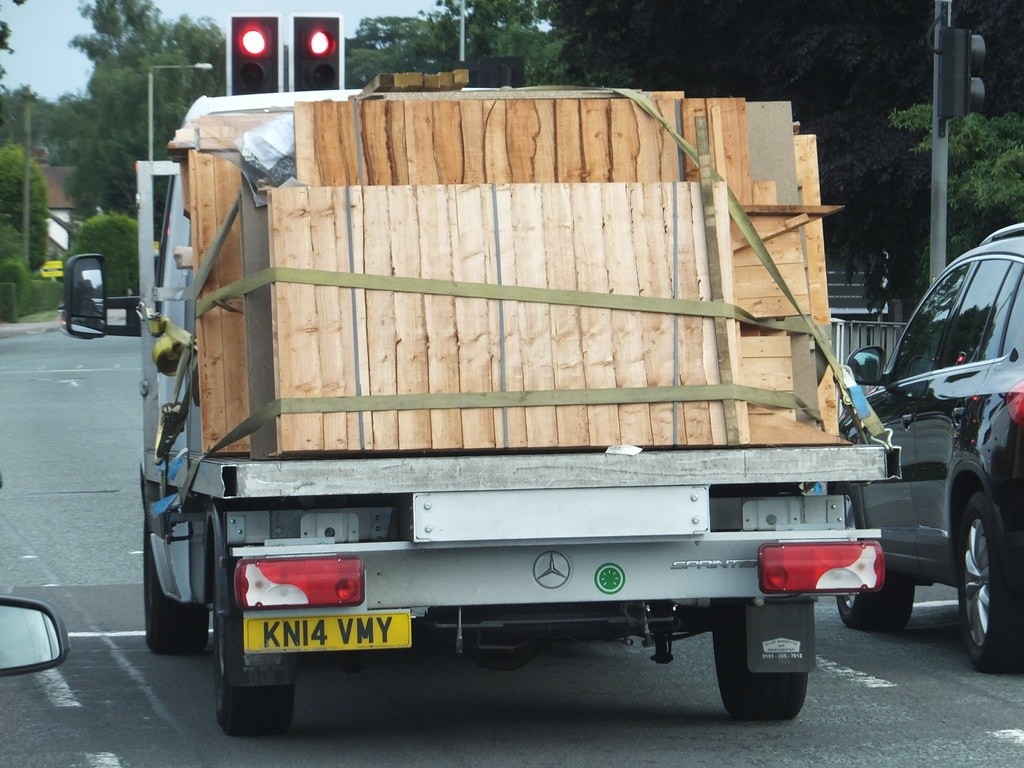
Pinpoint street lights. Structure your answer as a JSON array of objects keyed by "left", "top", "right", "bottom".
[{"left": 146, "top": 60, "right": 214, "bottom": 160}]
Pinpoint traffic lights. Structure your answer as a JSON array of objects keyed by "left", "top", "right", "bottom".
[
  {"left": 290, "top": 16, "right": 347, "bottom": 96},
  {"left": 226, "top": 17, "right": 282, "bottom": 97},
  {"left": 932, "top": 23, "right": 987, "bottom": 126}
]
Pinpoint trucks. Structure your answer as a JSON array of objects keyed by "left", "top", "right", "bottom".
[{"left": 57, "top": 103, "right": 911, "bottom": 742}]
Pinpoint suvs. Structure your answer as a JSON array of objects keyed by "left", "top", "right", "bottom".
[{"left": 818, "top": 219, "right": 1024, "bottom": 677}]
